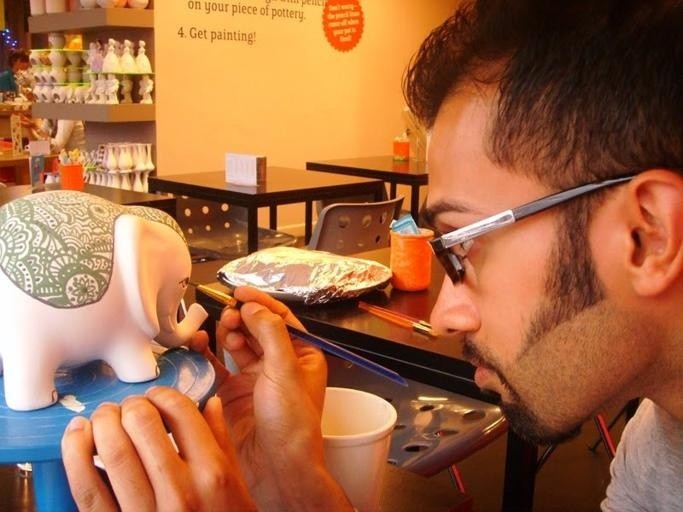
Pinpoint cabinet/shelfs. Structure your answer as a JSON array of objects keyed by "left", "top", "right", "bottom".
[{"left": 20, "top": 9, "right": 154, "bottom": 121}]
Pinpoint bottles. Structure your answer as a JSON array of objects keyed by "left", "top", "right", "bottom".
[{"left": 88, "top": 141, "right": 155, "bottom": 194}]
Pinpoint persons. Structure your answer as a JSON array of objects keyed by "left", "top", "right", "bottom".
[{"left": 59, "top": 1, "right": 683, "bottom": 512}]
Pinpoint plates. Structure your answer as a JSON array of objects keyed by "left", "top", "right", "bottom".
[{"left": 218, "top": 249, "right": 393, "bottom": 303}]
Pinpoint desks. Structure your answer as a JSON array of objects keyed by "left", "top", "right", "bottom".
[
  {"left": 0, "top": 183, "right": 177, "bottom": 219},
  {"left": 147, "top": 167, "right": 384, "bottom": 255},
  {"left": 194, "top": 246, "right": 538, "bottom": 512},
  {"left": 306, "top": 154, "right": 428, "bottom": 227}
]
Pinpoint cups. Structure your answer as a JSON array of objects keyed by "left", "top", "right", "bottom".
[
  {"left": 393, "top": 141, "right": 410, "bottom": 161},
  {"left": 390, "top": 227, "right": 435, "bottom": 291},
  {"left": 59, "top": 164, "right": 85, "bottom": 192},
  {"left": 320, "top": 387, "right": 397, "bottom": 512}
]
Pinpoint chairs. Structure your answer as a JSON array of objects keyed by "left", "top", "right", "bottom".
[
  {"left": 157, "top": 190, "right": 297, "bottom": 260},
  {"left": 305, "top": 195, "right": 406, "bottom": 256},
  {"left": 316, "top": 187, "right": 389, "bottom": 219},
  {"left": 324, "top": 354, "right": 506, "bottom": 497}
]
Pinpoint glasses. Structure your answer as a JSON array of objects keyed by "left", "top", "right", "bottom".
[{"left": 427, "top": 175, "right": 636, "bottom": 286}]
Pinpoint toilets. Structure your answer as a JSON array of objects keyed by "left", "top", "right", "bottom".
[{"left": 0, "top": 340, "right": 216, "bottom": 511}]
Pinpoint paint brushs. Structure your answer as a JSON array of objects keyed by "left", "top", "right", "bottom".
[{"left": 186, "top": 279, "right": 437, "bottom": 387}]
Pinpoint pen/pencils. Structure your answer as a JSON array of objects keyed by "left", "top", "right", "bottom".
[{"left": 58, "top": 148, "right": 83, "bottom": 164}]
